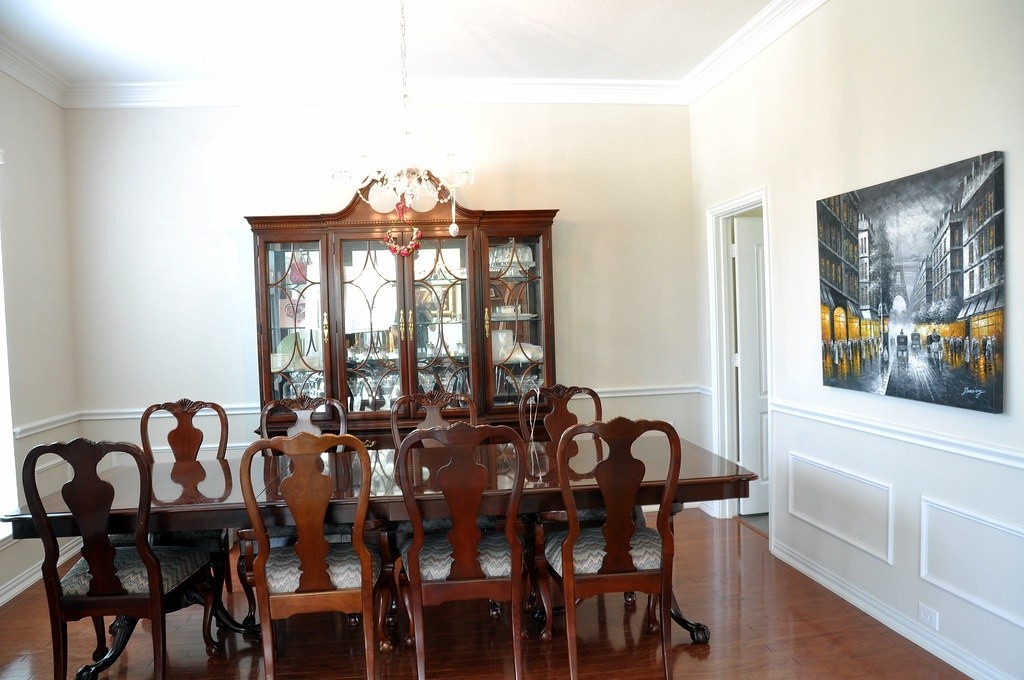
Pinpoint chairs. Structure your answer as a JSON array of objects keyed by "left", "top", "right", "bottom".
[{"left": 21, "top": 384, "right": 683, "bottom": 680}]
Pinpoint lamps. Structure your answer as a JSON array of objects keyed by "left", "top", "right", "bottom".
[{"left": 331, "top": 0, "right": 474, "bottom": 239}]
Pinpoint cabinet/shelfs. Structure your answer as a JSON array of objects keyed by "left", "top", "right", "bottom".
[{"left": 242, "top": 168, "right": 561, "bottom": 455}]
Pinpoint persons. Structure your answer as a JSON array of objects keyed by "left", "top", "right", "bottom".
[{"left": 391, "top": 287, "right": 437, "bottom": 352}]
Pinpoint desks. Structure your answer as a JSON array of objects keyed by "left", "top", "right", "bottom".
[{"left": 0, "top": 431, "right": 760, "bottom": 680}]
